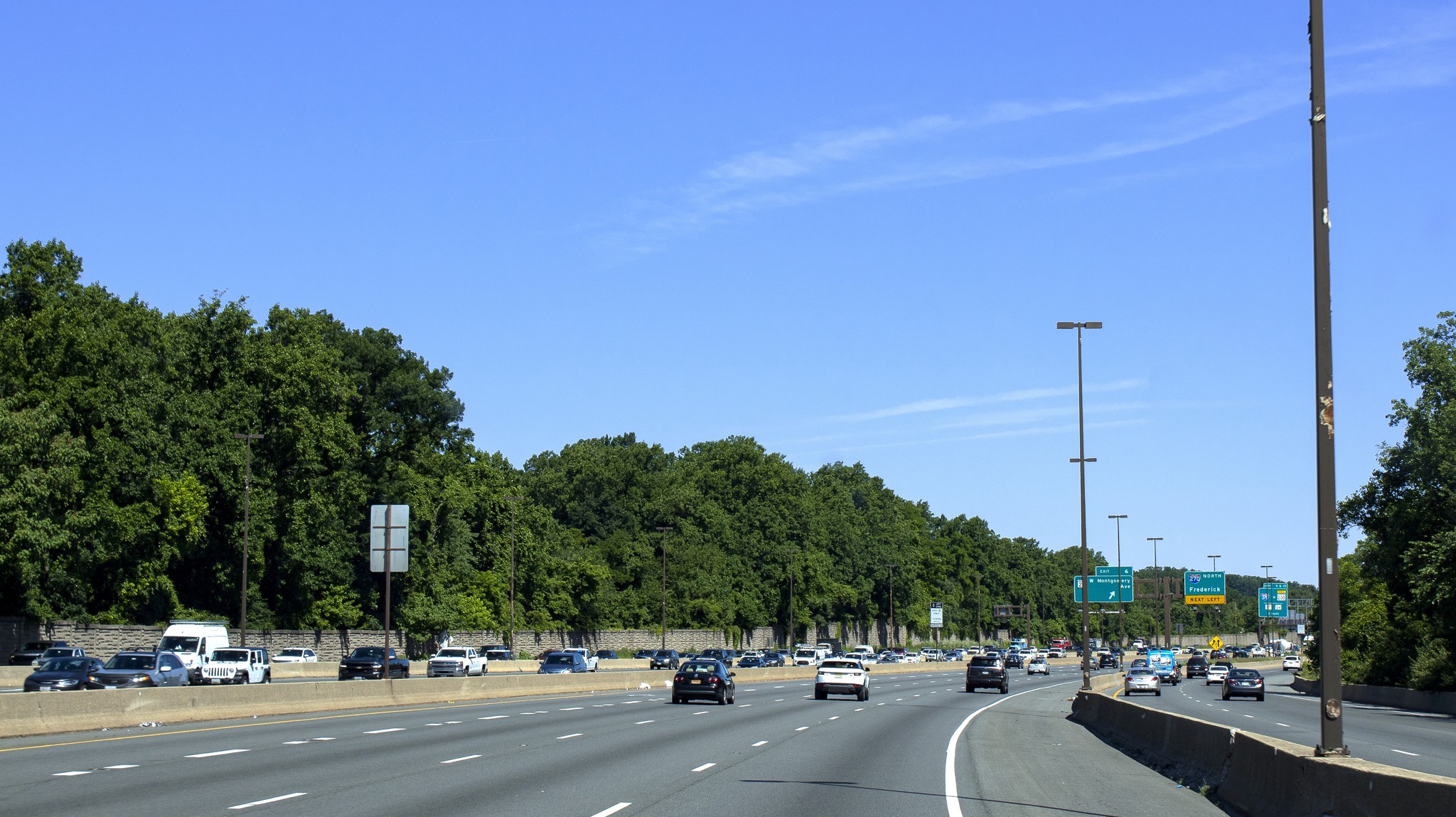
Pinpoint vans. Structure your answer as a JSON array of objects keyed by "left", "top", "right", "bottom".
[{"left": 152, "top": 619, "right": 230, "bottom": 686}]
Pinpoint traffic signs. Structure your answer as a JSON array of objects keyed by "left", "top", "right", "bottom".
[{"left": 1074, "top": 573, "right": 1135, "bottom": 605}]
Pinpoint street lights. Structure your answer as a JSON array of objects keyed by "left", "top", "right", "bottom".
[
  {"left": 1055, "top": 321, "right": 1103, "bottom": 691},
  {"left": 884, "top": 564, "right": 897, "bottom": 653},
  {"left": 785, "top": 549, "right": 797, "bottom": 657},
  {"left": 974, "top": 575, "right": 985, "bottom": 654},
  {"left": 655, "top": 526, "right": 673, "bottom": 649},
  {"left": 1207, "top": 555, "right": 1221, "bottom": 636},
  {"left": 502, "top": 496, "right": 524, "bottom": 661},
  {"left": 1146, "top": 537, "right": 1163, "bottom": 649},
  {"left": 1260, "top": 565, "right": 1276, "bottom": 657},
  {"left": 233, "top": 433, "right": 264, "bottom": 647},
  {"left": 1037, "top": 582, "right": 1047, "bottom": 649},
  {"left": 1108, "top": 515, "right": 1128, "bottom": 672}
]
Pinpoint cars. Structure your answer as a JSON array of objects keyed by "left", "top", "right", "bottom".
[
  {"left": 534, "top": 650, "right": 562, "bottom": 660},
  {"left": 537, "top": 652, "right": 587, "bottom": 674},
  {"left": 476, "top": 645, "right": 514, "bottom": 661},
  {"left": 270, "top": 647, "right": 317, "bottom": 663},
  {"left": 591, "top": 636, "right": 1315, "bottom": 706},
  {"left": 23, "top": 656, "right": 105, "bottom": 693}
]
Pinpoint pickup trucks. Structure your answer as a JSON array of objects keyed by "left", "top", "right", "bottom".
[
  {"left": 426, "top": 646, "right": 488, "bottom": 677},
  {"left": 337, "top": 647, "right": 410, "bottom": 681},
  {"left": 562, "top": 648, "right": 599, "bottom": 673}
]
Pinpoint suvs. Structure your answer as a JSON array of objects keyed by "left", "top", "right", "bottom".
[
  {"left": 200, "top": 646, "right": 270, "bottom": 685},
  {"left": 86, "top": 647, "right": 189, "bottom": 689},
  {"left": 9, "top": 640, "right": 66, "bottom": 667},
  {"left": 32, "top": 646, "right": 86, "bottom": 671}
]
